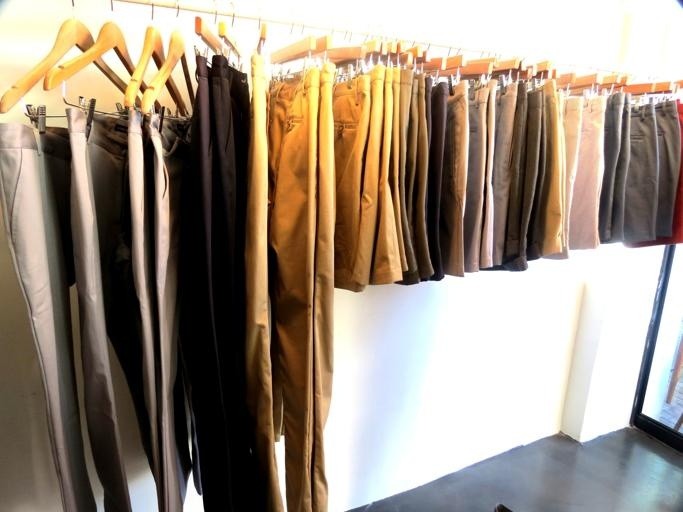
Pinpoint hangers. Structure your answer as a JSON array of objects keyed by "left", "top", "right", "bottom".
[{"left": 0, "top": 0, "right": 267, "bottom": 116}]
